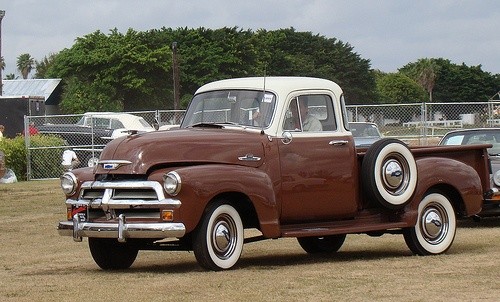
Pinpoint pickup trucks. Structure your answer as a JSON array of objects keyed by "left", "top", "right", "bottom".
[{"left": 56, "top": 77, "right": 493, "bottom": 272}]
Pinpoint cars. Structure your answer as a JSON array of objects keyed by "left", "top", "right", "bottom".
[
  {"left": 347, "top": 122, "right": 383, "bottom": 152},
  {"left": 37, "top": 112, "right": 181, "bottom": 168},
  {"left": 437, "top": 128, "right": 500, "bottom": 219}
]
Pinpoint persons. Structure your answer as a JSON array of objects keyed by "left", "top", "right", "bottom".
[
  {"left": 0, "top": 125, "right": 5, "bottom": 141},
  {"left": 29, "top": 122, "right": 37, "bottom": 134},
  {"left": 254, "top": 96, "right": 322, "bottom": 131}
]
